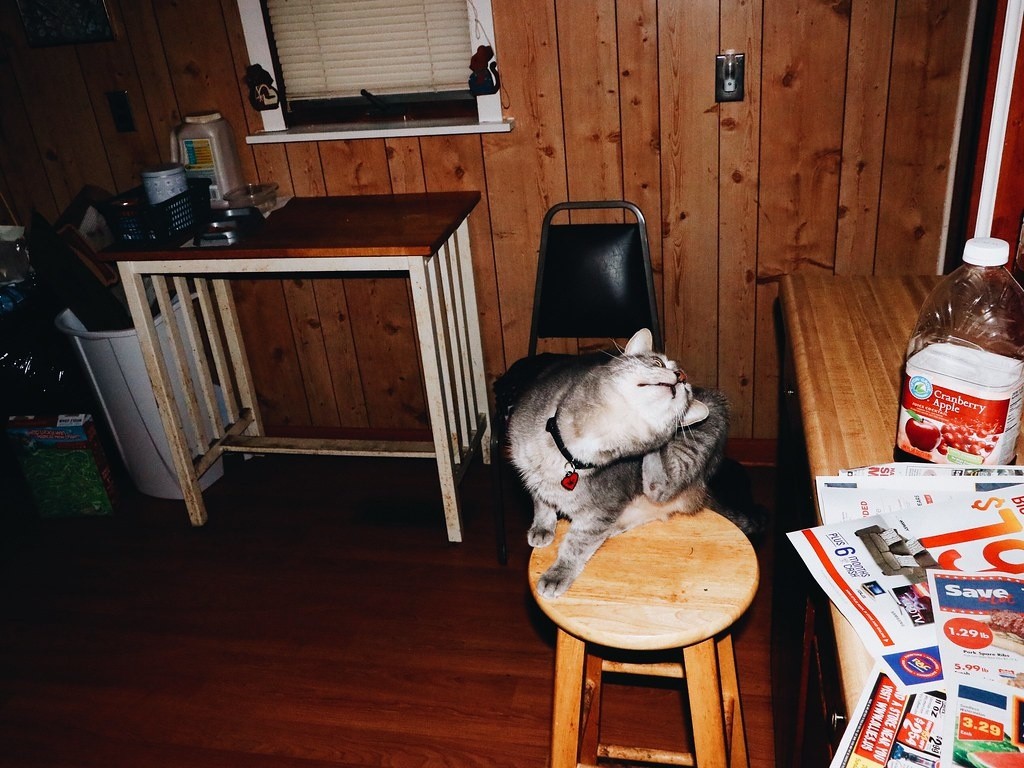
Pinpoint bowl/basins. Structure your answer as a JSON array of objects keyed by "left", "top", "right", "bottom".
[{"left": 223, "top": 181, "right": 281, "bottom": 213}]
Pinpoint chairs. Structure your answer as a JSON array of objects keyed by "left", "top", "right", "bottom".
[{"left": 488, "top": 200, "right": 663, "bottom": 563}]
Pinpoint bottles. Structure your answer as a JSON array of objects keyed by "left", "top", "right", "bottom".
[{"left": 892, "top": 237, "right": 1024, "bottom": 467}]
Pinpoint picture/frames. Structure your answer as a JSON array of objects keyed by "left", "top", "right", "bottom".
[{"left": 16, "top": 0, "right": 121, "bottom": 49}]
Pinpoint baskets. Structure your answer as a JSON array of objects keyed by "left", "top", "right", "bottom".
[{"left": 100, "top": 177, "right": 216, "bottom": 251}]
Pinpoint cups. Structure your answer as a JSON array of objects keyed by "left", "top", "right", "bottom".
[{"left": 141, "top": 163, "right": 195, "bottom": 235}]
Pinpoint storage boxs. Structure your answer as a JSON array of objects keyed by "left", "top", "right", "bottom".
[
  {"left": 95, "top": 177, "right": 213, "bottom": 248},
  {"left": 7, "top": 413, "right": 122, "bottom": 517}
]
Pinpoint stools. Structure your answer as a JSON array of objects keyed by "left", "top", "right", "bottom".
[{"left": 528, "top": 501, "right": 760, "bottom": 768}]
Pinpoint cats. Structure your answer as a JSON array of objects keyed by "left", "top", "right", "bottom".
[{"left": 493, "top": 327, "right": 731, "bottom": 600}]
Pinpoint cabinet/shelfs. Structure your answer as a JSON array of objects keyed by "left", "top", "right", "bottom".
[{"left": 778, "top": 271, "right": 1024, "bottom": 768}]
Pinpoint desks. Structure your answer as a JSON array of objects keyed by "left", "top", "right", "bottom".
[{"left": 98, "top": 190, "right": 492, "bottom": 545}]
